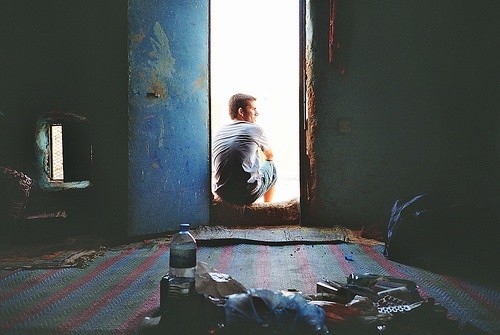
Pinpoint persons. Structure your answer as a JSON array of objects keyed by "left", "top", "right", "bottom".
[{"left": 211, "top": 93, "right": 277, "bottom": 205}]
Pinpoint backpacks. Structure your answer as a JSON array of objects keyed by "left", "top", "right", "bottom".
[{"left": 384, "top": 191, "right": 446, "bottom": 263}]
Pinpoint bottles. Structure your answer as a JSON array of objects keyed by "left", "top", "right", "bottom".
[{"left": 167, "top": 224, "right": 197, "bottom": 306}]
